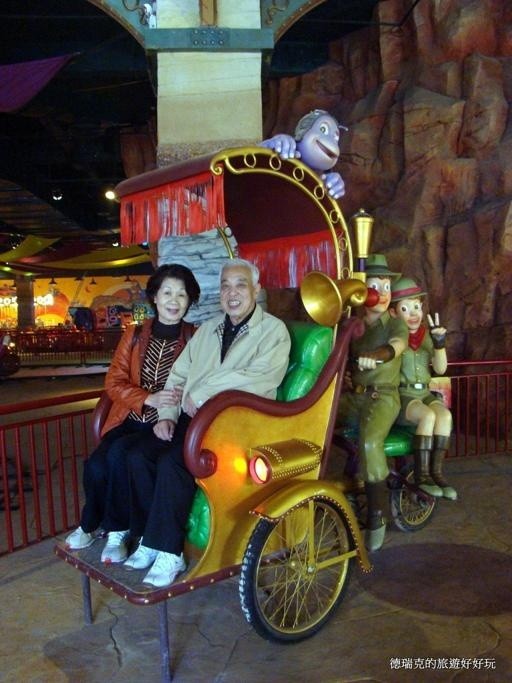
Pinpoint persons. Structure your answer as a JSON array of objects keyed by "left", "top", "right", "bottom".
[
  {"left": 121, "top": 257, "right": 294, "bottom": 590},
  {"left": 388, "top": 276, "right": 458, "bottom": 507},
  {"left": 333, "top": 250, "right": 409, "bottom": 559},
  {"left": 63, "top": 263, "right": 201, "bottom": 568}
]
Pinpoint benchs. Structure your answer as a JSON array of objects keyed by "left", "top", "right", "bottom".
[{"left": 106, "top": 322, "right": 333, "bottom": 548}]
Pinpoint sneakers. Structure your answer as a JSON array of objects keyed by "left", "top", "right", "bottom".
[
  {"left": 100, "top": 529, "right": 130, "bottom": 564},
  {"left": 143, "top": 551, "right": 187, "bottom": 589},
  {"left": 65, "top": 525, "right": 106, "bottom": 550},
  {"left": 124, "top": 535, "right": 161, "bottom": 570}
]
